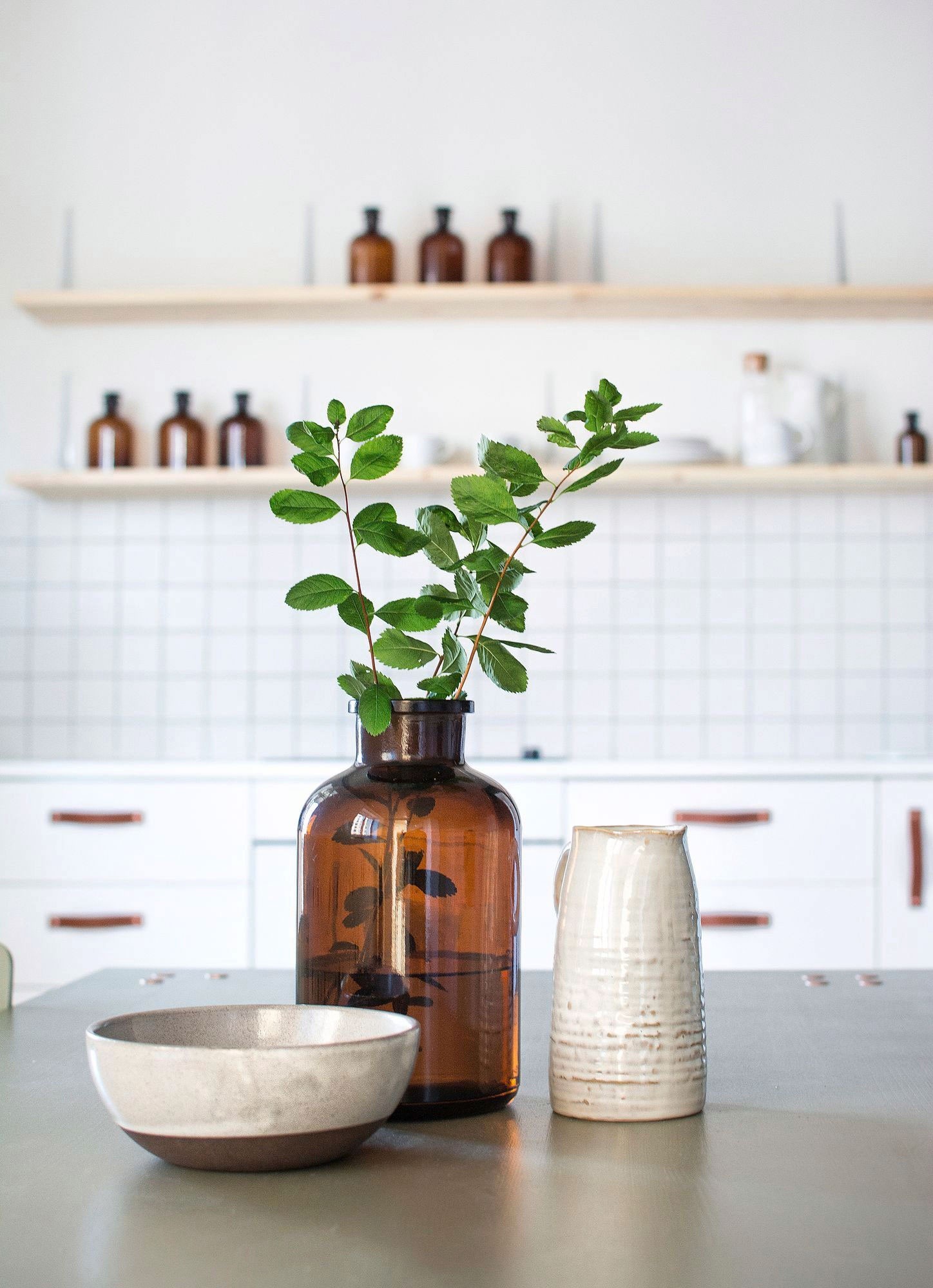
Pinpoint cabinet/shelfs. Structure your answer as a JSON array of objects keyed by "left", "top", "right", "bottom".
[
  {"left": 15, "top": 284, "right": 933, "bottom": 500},
  {"left": 0, "top": 774, "right": 933, "bottom": 1010}
]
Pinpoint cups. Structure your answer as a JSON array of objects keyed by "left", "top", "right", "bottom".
[{"left": 546, "top": 822, "right": 710, "bottom": 1120}]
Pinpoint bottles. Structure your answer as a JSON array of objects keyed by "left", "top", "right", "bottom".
[
  {"left": 157, "top": 392, "right": 205, "bottom": 465},
  {"left": 86, "top": 393, "right": 133, "bottom": 467},
  {"left": 350, "top": 209, "right": 394, "bottom": 281},
  {"left": 218, "top": 393, "right": 262, "bottom": 465},
  {"left": 485, "top": 209, "right": 534, "bottom": 283},
  {"left": 738, "top": 353, "right": 772, "bottom": 460},
  {"left": 895, "top": 411, "right": 930, "bottom": 466},
  {"left": 419, "top": 206, "right": 464, "bottom": 282}
]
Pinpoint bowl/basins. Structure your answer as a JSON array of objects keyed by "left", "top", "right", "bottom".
[
  {"left": 83, "top": 1002, "right": 420, "bottom": 1172},
  {"left": 740, "top": 419, "right": 805, "bottom": 467}
]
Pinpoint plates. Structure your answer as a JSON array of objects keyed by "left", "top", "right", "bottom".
[
  {"left": 342, "top": 437, "right": 446, "bottom": 467},
  {"left": 603, "top": 439, "right": 715, "bottom": 465}
]
